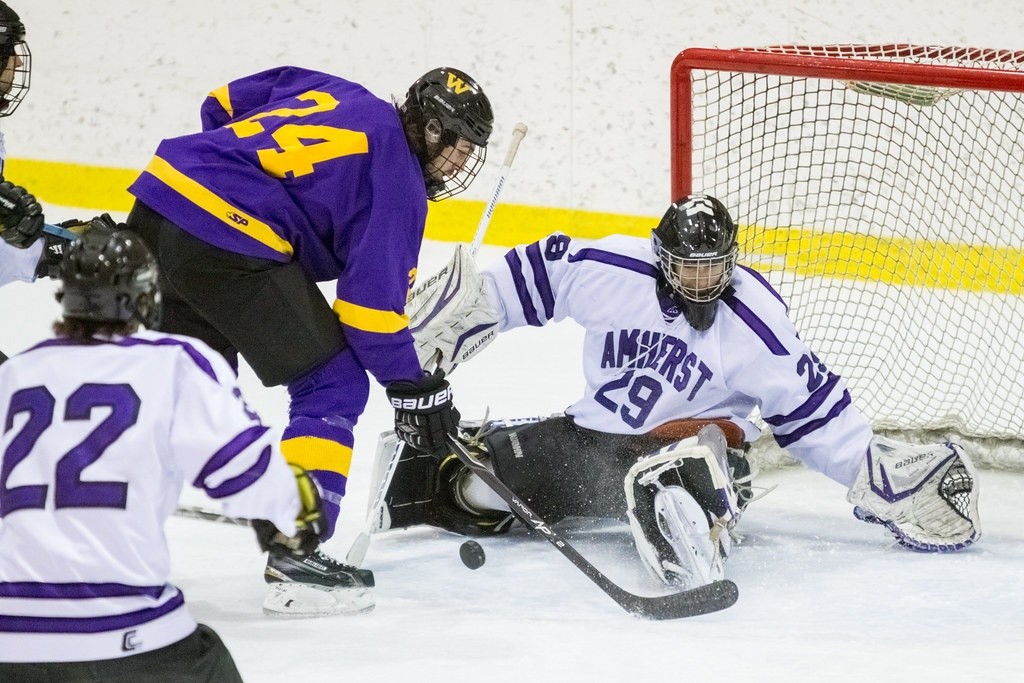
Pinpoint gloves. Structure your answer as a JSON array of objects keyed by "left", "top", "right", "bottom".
[
  {"left": 0, "top": 175, "right": 45, "bottom": 249},
  {"left": 44, "top": 213, "right": 129, "bottom": 258},
  {"left": 386, "top": 366, "right": 462, "bottom": 461}
]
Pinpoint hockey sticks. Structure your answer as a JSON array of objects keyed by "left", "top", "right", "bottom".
[
  {"left": 172, "top": 508, "right": 252, "bottom": 528},
  {"left": 445, "top": 433, "right": 740, "bottom": 621},
  {"left": 40, "top": 221, "right": 82, "bottom": 240},
  {"left": 342, "top": 121, "right": 530, "bottom": 569}
]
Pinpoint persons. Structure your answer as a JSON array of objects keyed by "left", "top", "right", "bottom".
[
  {"left": 122, "top": 62, "right": 496, "bottom": 620},
  {"left": 0, "top": 222, "right": 329, "bottom": 683},
  {"left": 364, "top": 191, "right": 928, "bottom": 599},
  {"left": 0, "top": 0, "right": 128, "bottom": 294}
]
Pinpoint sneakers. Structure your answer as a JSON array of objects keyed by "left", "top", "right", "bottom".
[
  {"left": 262, "top": 543, "right": 377, "bottom": 615},
  {"left": 655, "top": 485, "right": 723, "bottom": 589}
]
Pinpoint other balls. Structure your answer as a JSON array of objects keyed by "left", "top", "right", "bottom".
[{"left": 458, "top": 539, "right": 486, "bottom": 570}]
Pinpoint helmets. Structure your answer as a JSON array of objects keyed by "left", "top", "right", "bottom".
[
  {"left": 0, "top": 1, "right": 33, "bottom": 117},
  {"left": 60, "top": 228, "right": 164, "bottom": 331},
  {"left": 650, "top": 193, "right": 740, "bottom": 302},
  {"left": 407, "top": 66, "right": 495, "bottom": 202}
]
